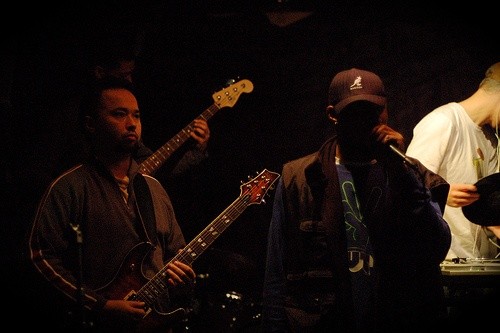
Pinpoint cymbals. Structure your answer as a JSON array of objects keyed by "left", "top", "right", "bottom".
[{"left": 462, "top": 172, "right": 500, "bottom": 226}]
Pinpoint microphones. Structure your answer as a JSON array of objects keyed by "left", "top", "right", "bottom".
[{"left": 388, "top": 143, "right": 418, "bottom": 171}]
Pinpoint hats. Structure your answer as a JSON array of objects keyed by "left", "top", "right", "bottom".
[{"left": 328, "top": 67, "right": 385, "bottom": 116}]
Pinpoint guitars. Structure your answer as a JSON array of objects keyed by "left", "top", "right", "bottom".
[
  {"left": 74, "top": 167, "right": 280, "bottom": 333},
  {"left": 140, "top": 76, "right": 255, "bottom": 174}
]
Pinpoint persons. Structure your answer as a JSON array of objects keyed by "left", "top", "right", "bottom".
[
  {"left": 23, "top": 84, "right": 195, "bottom": 331},
  {"left": 261, "top": 66, "right": 451, "bottom": 330},
  {"left": 404, "top": 61, "right": 496, "bottom": 262},
  {"left": 31, "top": 33, "right": 211, "bottom": 231}
]
